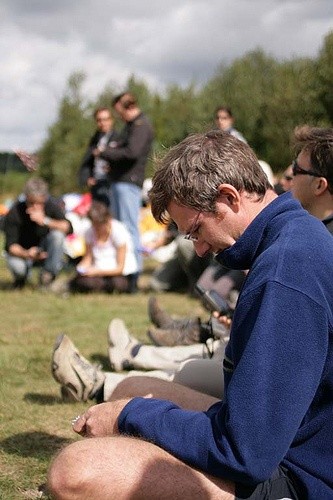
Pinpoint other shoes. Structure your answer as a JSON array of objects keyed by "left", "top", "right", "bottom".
[
  {"left": 13, "top": 277, "right": 25, "bottom": 289},
  {"left": 41, "top": 273, "right": 54, "bottom": 284}
]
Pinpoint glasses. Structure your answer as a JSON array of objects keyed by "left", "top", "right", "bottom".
[
  {"left": 292, "top": 160, "right": 322, "bottom": 178},
  {"left": 184, "top": 208, "right": 204, "bottom": 242},
  {"left": 26, "top": 199, "right": 48, "bottom": 206}
]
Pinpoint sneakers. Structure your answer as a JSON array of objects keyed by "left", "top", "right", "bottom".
[
  {"left": 51, "top": 334, "right": 105, "bottom": 402},
  {"left": 108, "top": 318, "right": 142, "bottom": 372},
  {"left": 149, "top": 298, "right": 201, "bottom": 329},
  {"left": 147, "top": 326, "right": 214, "bottom": 347}
]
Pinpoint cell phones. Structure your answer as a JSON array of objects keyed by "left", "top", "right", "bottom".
[{"left": 194, "top": 284, "right": 233, "bottom": 319}]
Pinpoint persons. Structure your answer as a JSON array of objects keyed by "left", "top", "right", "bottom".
[
  {"left": 49, "top": 130, "right": 333, "bottom": 500},
  {"left": 4, "top": 177, "right": 73, "bottom": 292},
  {"left": 52, "top": 91, "right": 333, "bottom": 402}
]
